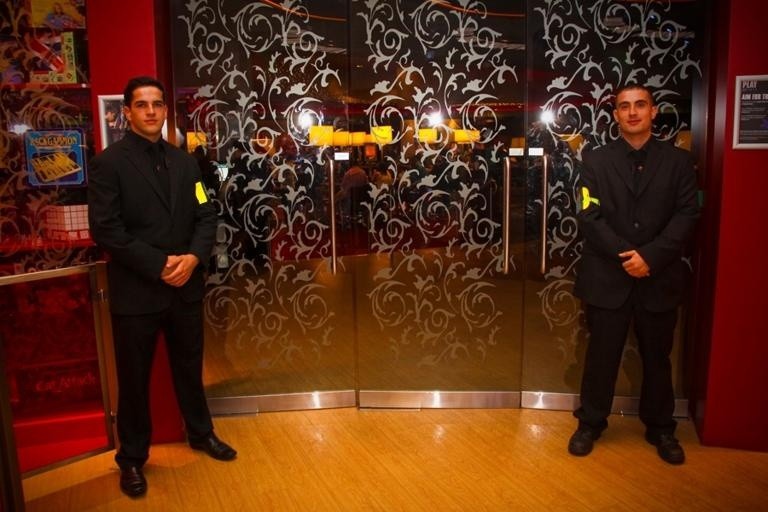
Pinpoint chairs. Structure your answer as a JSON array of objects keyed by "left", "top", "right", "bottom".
[{"left": 338, "top": 184, "right": 373, "bottom": 232}]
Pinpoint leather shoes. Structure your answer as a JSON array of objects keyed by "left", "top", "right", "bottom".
[
  {"left": 566, "top": 414, "right": 609, "bottom": 455},
  {"left": 187, "top": 429, "right": 239, "bottom": 462},
  {"left": 643, "top": 419, "right": 689, "bottom": 465},
  {"left": 119, "top": 464, "right": 149, "bottom": 500}
]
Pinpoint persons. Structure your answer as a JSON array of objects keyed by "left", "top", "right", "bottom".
[
  {"left": 87, "top": 74, "right": 237, "bottom": 497},
  {"left": 566, "top": 81, "right": 703, "bottom": 464}
]
